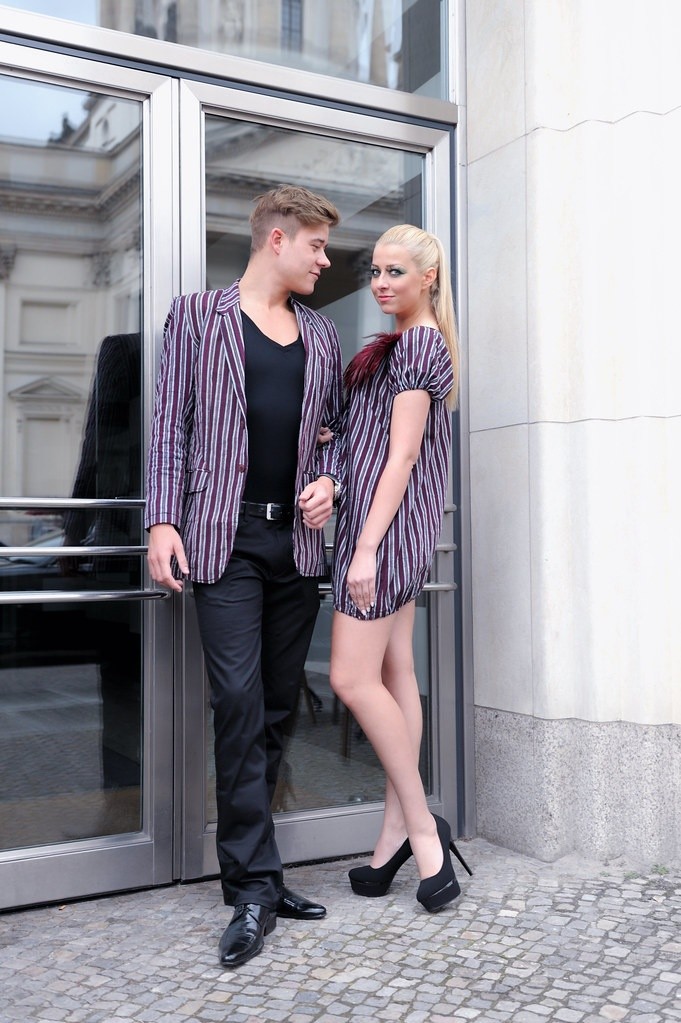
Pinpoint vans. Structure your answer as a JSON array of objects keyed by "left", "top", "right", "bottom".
[{"left": 0, "top": 516, "right": 63, "bottom": 546}]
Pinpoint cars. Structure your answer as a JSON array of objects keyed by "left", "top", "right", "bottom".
[{"left": 0, "top": 526, "right": 96, "bottom": 591}]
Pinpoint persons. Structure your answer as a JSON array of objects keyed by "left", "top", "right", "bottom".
[
  {"left": 317, "top": 223, "right": 473, "bottom": 913},
  {"left": 147, "top": 186, "right": 348, "bottom": 967}
]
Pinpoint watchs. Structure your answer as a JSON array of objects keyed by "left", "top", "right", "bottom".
[{"left": 318, "top": 473, "right": 343, "bottom": 500}]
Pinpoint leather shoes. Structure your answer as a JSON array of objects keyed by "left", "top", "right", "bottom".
[
  {"left": 273, "top": 886, "right": 326, "bottom": 920},
  {"left": 218, "top": 901, "right": 276, "bottom": 969}
]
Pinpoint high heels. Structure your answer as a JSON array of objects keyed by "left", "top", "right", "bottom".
[
  {"left": 348, "top": 838, "right": 413, "bottom": 897},
  {"left": 416, "top": 810, "right": 472, "bottom": 911}
]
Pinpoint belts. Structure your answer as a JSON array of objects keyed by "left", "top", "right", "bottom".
[{"left": 237, "top": 500, "right": 296, "bottom": 522}]
